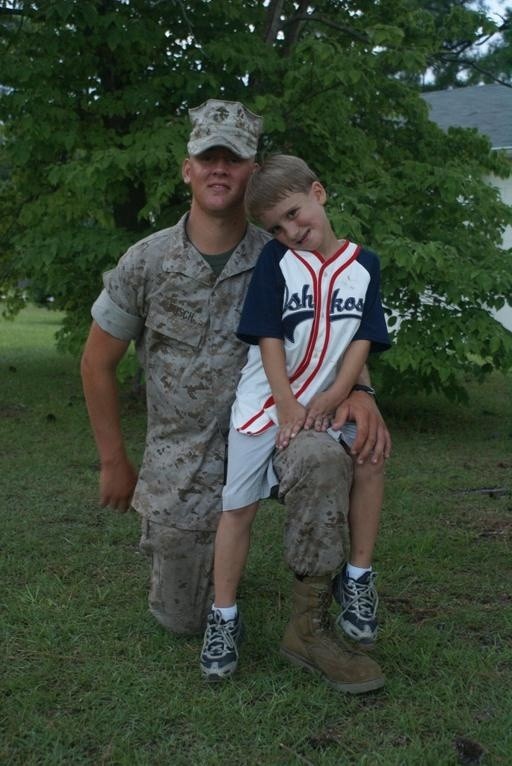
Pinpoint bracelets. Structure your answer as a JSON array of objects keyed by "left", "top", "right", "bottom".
[{"left": 351, "top": 384, "right": 379, "bottom": 403}]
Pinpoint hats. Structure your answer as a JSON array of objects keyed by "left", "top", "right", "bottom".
[{"left": 187, "top": 99, "right": 264, "bottom": 159}]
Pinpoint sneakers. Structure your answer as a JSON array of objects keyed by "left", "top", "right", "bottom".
[
  {"left": 199, "top": 607, "right": 242, "bottom": 682},
  {"left": 332, "top": 561, "right": 379, "bottom": 644}
]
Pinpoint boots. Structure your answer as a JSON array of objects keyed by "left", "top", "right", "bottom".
[{"left": 280, "top": 577, "right": 386, "bottom": 695}]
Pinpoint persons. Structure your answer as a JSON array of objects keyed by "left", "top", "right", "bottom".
[
  {"left": 197, "top": 154, "right": 387, "bottom": 683},
  {"left": 81, "top": 100, "right": 392, "bottom": 697}
]
